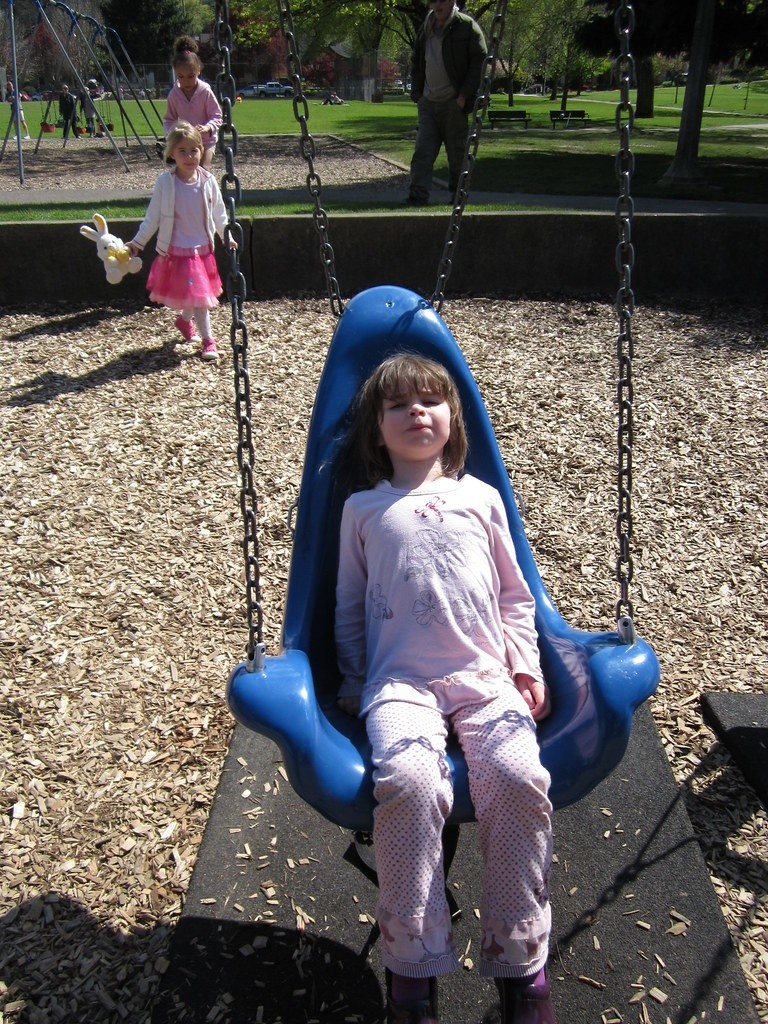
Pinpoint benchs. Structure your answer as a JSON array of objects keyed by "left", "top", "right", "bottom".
[
  {"left": 550, "top": 110, "right": 591, "bottom": 129},
  {"left": 487, "top": 111, "right": 531, "bottom": 129}
]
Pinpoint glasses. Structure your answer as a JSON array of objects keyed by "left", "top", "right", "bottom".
[{"left": 431, "top": 0, "right": 446, "bottom": 3}]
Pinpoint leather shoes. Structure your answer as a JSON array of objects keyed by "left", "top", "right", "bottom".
[
  {"left": 493, "top": 963, "right": 555, "bottom": 1024},
  {"left": 383, "top": 965, "right": 438, "bottom": 1024}
]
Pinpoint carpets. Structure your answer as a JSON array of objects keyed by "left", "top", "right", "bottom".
[{"left": 152, "top": 700, "right": 762, "bottom": 1024}]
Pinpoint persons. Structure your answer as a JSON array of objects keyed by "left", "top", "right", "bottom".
[
  {"left": 60, "top": 84, "right": 81, "bottom": 139},
  {"left": 124, "top": 121, "right": 239, "bottom": 360},
  {"left": 162, "top": 35, "right": 223, "bottom": 172},
  {"left": 6, "top": 81, "right": 31, "bottom": 142},
  {"left": 78, "top": 86, "right": 101, "bottom": 138},
  {"left": 399, "top": 1, "right": 488, "bottom": 205},
  {"left": 324, "top": 91, "right": 347, "bottom": 105},
  {"left": 323, "top": 351, "right": 559, "bottom": 1024}
]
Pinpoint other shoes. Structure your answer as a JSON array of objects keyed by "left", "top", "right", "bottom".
[
  {"left": 76, "top": 135, "right": 81, "bottom": 138},
  {"left": 24, "top": 136, "right": 30, "bottom": 140},
  {"left": 13, "top": 135, "right": 17, "bottom": 140},
  {"left": 61, "top": 136, "right": 69, "bottom": 140}
]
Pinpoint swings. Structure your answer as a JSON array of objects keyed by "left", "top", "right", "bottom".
[
  {"left": 33, "top": 1, "right": 115, "bottom": 135},
  {"left": 204, "top": 0, "right": 661, "bottom": 827}
]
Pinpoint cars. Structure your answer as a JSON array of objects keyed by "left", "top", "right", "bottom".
[
  {"left": 382, "top": 78, "right": 413, "bottom": 95},
  {"left": 233, "top": 84, "right": 265, "bottom": 98},
  {"left": 674, "top": 73, "right": 689, "bottom": 84}
]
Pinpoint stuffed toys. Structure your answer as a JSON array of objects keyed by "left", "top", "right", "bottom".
[{"left": 80, "top": 213, "right": 142, "bottom": 285}]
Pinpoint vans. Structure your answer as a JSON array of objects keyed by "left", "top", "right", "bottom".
[{"left": 7, "top": 88, "right": 157, "bottom": 101}]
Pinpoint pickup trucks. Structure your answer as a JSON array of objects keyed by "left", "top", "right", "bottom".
[{"left": 254, "top": 82, "right": 294, "bottom": 98}]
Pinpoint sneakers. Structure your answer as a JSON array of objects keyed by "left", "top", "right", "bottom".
[
  {"left": 202, "top": 337, "right": 219, "bottom": 360},
  {"left": 173, "top": 315, "right": 202, "bottom": 344}
]
